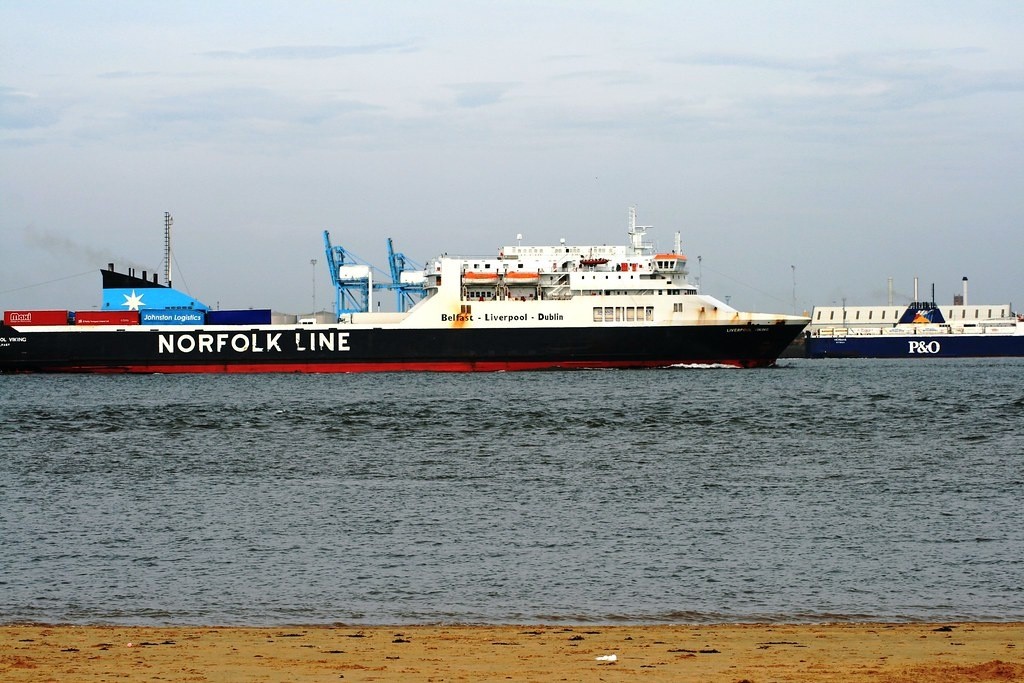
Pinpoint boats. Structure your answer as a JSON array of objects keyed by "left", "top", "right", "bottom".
[
  {"left": 1, "top": 205, "right": 812, "bottom": 367},
  {"left": 800, "top": 277, "right": 1024, "bottom": 358}
]
